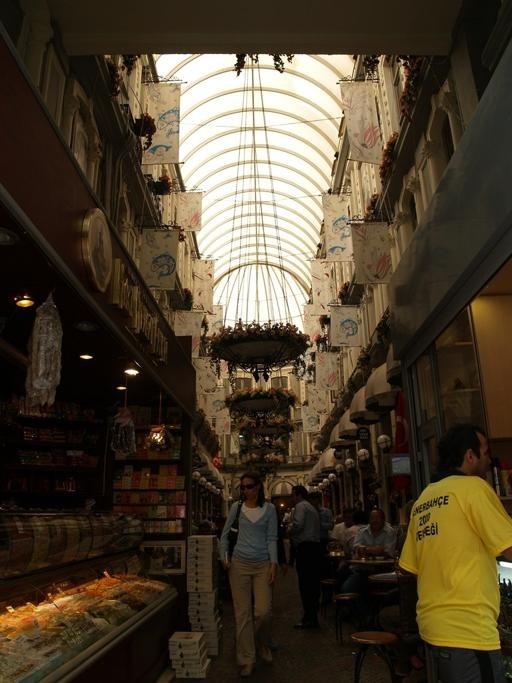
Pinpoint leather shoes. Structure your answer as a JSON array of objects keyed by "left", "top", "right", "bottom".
[{"left": 294, "top": 622, "right": 320, "bottom": 629}]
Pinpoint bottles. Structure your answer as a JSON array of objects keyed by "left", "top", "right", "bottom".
[{"left": 394, "top": 556, "right": 400, "bottom": 573}]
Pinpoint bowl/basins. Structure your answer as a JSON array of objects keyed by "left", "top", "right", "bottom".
[{"left": 376, "top": 556, "right": 385, "bottom": 560}]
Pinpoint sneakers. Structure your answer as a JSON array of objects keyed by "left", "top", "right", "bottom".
[
  {"left": 241, "top": 663, "right": 253, "bottom": 676},
  {"left": 258, "top": 647, "right": 272, "bottom": 662},
  {"left": 268, "top": 641, "right": 280, "bottom": 650}
]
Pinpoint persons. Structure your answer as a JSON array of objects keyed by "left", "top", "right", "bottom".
[
  {"left": 284, "top": 483, "right": 396, "bottom": 630},
  {"left": 395, "top": 424, "right": 512, "bottom": 683},
  {"left": 219, "top": 472, "right": 280, "bottom": 680}
]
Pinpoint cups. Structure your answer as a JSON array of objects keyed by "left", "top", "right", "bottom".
[{"left": 358, "top": 545, "right": 367, "bottom": 559}]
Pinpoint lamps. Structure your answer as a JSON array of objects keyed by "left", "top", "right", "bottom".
[
  {"left": 14, "top": 293, "right": 171, "bottom": 452},
  {"left": 305, "top": 435, "right": 392, "bottom": 493}
]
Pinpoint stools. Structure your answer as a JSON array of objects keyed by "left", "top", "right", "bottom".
[{"left": 318, "top": 575, "right": 413, "bottom": 682}]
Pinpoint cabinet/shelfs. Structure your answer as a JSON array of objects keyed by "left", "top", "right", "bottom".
[{"left": 0, "top": 409, "right": 224, "bottom": 682}]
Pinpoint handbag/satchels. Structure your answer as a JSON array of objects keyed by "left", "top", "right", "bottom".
[{"left": 227, "top": 518, "right": 239, "bottom": 557}]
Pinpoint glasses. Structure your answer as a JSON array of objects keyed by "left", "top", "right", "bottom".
[{"left": 240, "top": 482, "right": 258, "bottom": 490}]
